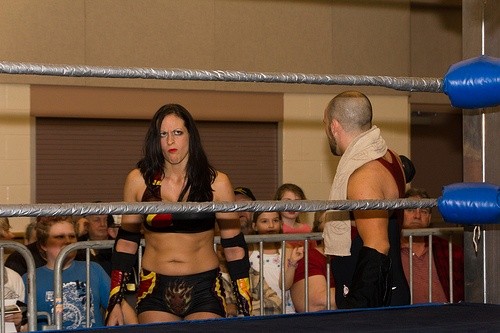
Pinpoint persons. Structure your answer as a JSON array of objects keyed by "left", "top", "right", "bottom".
[
  {"left": 400, "top": 188, "right": 464, "bottom": 306},
  {"left": 103, "top": 103, "right": 254, "bottom": 328},
  {"left": 322, "top": 89, "right": 412, "bottom": 310},
  {"left": 0, "top": 200, "right": 123, "bottom": 333},
  {"left": 215, "top": 183, "right": 338, "bottom": 318}
]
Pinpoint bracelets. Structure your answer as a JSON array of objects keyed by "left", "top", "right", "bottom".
[{"left": 287, "top": 257, "right": 298, "bottom": 269}]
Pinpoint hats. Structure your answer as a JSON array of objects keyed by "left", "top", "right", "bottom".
[{"left": 233, "top": 187, "right": 256, "bottom": 202}]
